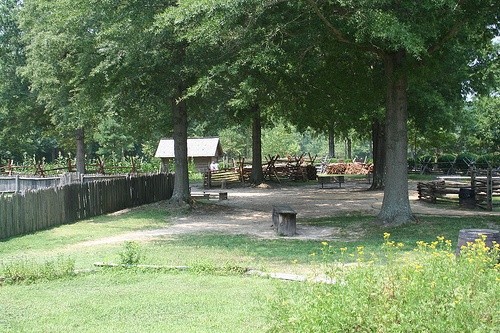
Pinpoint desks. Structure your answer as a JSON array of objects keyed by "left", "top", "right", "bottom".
[{"left": 318, "top": 174, "right": 371, "bottom": 189}]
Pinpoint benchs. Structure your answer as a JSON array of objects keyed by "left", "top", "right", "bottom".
[{"left": 272, "top": 203, "right": 297, "bottom": 236}]
